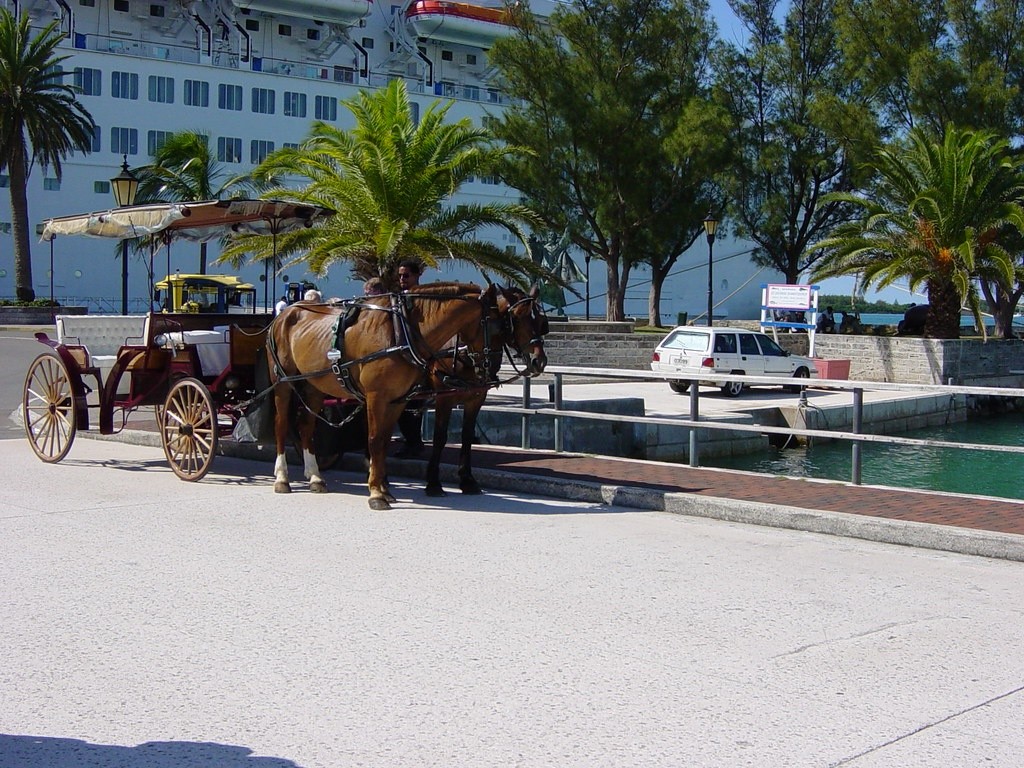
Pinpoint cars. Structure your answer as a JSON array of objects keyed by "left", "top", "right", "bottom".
[{"left": 649, "top": 324, "right": 820, "bottom": 398}]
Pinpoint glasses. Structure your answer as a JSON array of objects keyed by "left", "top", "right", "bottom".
[{"left": 398, "top": 272, "right": 410, "bottom": 280}]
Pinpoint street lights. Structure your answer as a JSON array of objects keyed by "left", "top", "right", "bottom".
[
  {"left": 703, "top": 207, "right": 719, "bottom": 328},
  {"left": 584, "top": 246, "right": 593, "bottom": 322},
  {"left": 111, "top": 154, "right": 139, "bottom": 318}
]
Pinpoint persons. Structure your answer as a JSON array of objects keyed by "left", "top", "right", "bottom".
[
  {"left": 304, "top": 290, "right": 322, "bottom": 303},
  {"left": 276, "top": 296, "right": 288, "bottom": 316},
  {"left": 160, "top": 291, "right": 245, "bottom": 314},
  {"left": 364, "top": 260, "right": 437, "bottom": 459},
  {"left": 775, "top": 302, "right": 918, "bottom": 334}
]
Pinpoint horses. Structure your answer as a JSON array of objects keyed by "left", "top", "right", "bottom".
[{"left": 264, "top": 279, "right": 550, "bottom": 510}]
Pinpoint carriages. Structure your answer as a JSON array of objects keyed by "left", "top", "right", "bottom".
[{"left": 21, "top": 193, "right": 550, "bottom": 516}]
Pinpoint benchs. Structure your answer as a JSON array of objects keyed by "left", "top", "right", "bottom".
[
  {"left": 55, "top": 315, "right": 150, "bottom": 368},
  {"left": 164, "top": 325, "right": 230, "bottom": 374}
]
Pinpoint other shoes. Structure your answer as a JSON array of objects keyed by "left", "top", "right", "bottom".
[{"left": 392, "top": 443, "right": 425, "bottom": 459}]
